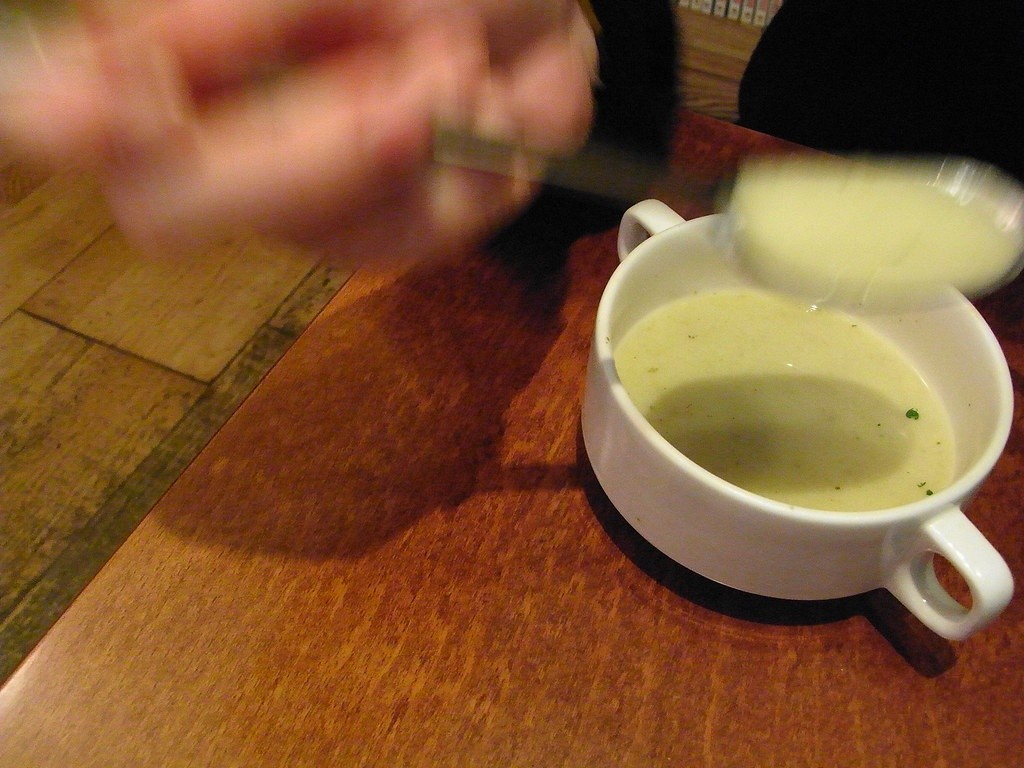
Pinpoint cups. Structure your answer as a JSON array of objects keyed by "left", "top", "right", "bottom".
[{"left": 579, "top": 197, "right": 1013, "bottom": 642}]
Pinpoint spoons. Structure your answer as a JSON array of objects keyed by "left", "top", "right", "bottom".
[{"left": 250, "top": 53, "right": 1024, "bottom": 311}]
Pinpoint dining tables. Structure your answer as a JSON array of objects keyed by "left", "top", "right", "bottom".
[{"left": 0, "top": 106, "right": 1023, "bottom": 768}]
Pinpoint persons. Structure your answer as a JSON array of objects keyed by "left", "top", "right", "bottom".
[{"left": 0, "top": 0, "right": 1024, "bottom": 328}]
